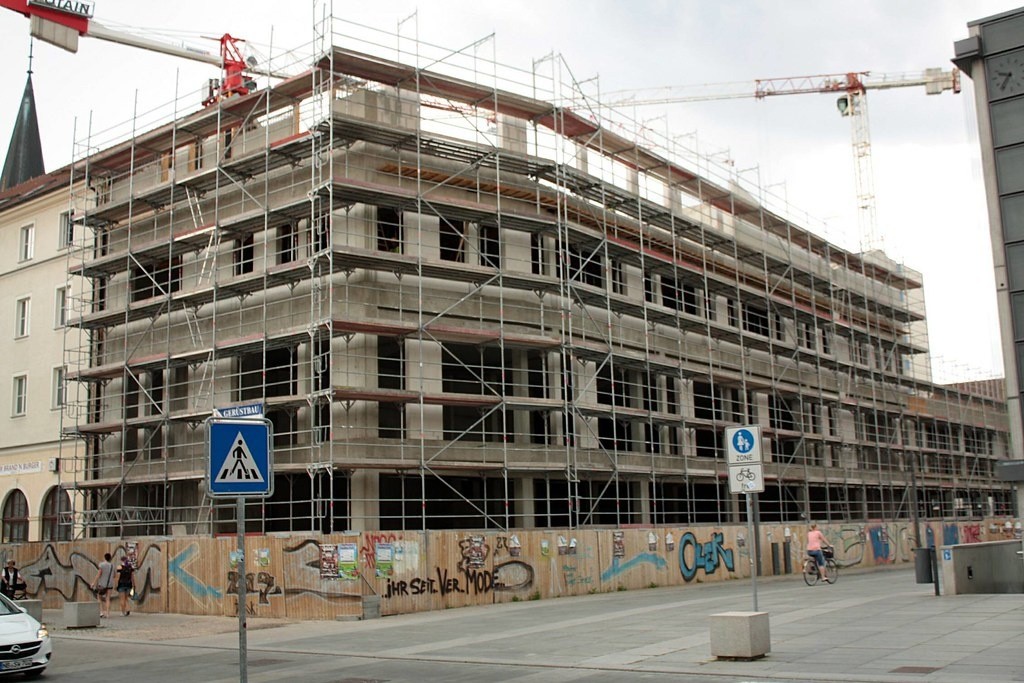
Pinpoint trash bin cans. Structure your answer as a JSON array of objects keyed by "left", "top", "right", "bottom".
[{"left": 910, "top": 548, "right": 933, "bottom": 584}]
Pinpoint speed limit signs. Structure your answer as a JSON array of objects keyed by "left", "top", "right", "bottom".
[{"left": 208, "top": 420, "right": 270, "bottom": 495}]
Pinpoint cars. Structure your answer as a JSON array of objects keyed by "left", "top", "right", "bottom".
[{"left": 0, "top": 592, "right": 52, "bottom": 679}]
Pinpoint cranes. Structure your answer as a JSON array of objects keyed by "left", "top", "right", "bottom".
[{"left": 420, "top": 66, "right": 962, "bottom": 249}]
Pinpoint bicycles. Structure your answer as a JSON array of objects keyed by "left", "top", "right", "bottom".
[{"left": 802, "top": 545, "right": 838, "bottom": 586}]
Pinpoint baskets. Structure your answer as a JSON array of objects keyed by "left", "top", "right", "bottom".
[{"left": 823, "top": 550, "right": 834, "bottom": 558}]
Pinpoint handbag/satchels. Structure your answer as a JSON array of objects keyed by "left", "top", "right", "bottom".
[
  {"left": 131, "top": 587, "right": 140, "bottom": 600},
  {"left": 129, "top": 587, "right": 135, "bottom": 597},
  {"left": 97, "top": 585, "right": 107, "bottom": 595}
]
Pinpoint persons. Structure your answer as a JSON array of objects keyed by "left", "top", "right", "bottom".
[
  {"left": 91, "top": 553, "right": 116, "bottom": 618},
  {"left": 0, "top": 559, "right": 23, "bottom": 599},
  {"left": 806, "top": 524, "right": 834, "bottom": 582},
  {"left": 115, "top": 556, "right": 135, "bottom": 617}
]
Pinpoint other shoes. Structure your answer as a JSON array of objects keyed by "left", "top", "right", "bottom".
[{"left": 125, "top": 611, "right": 130, "bottom": 616}]
